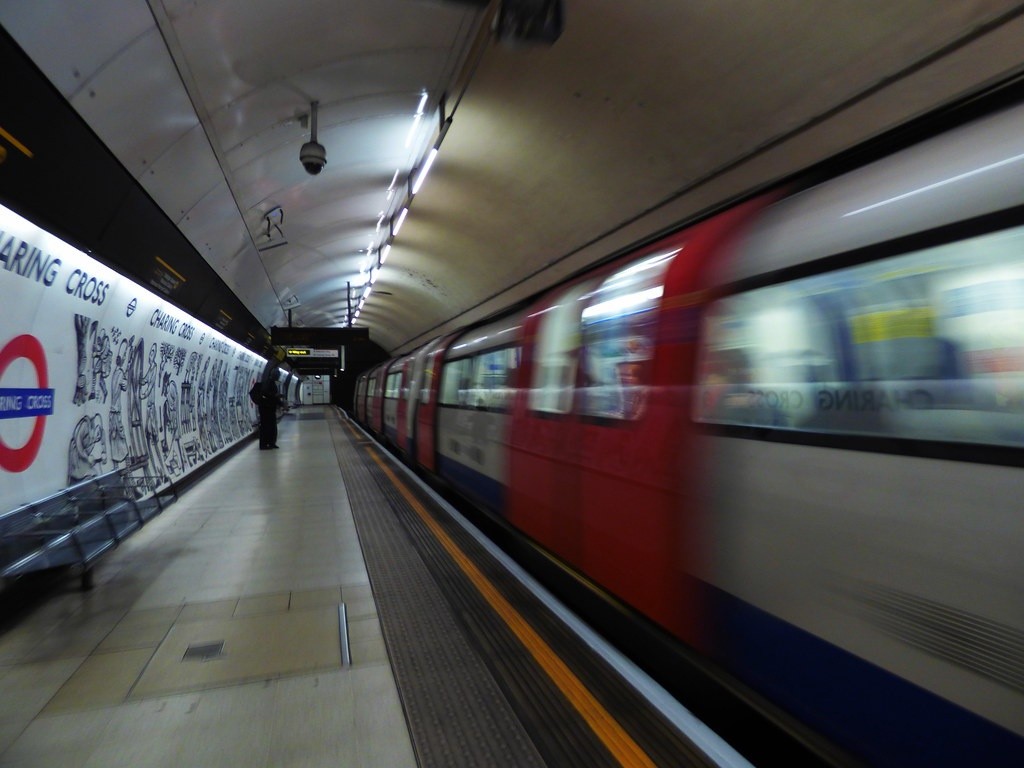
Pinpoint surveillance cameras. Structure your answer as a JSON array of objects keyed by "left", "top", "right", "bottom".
[{"left": 299, "top": 142, "right": 327, "bottom": 176}]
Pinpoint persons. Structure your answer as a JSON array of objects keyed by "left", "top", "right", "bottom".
[
  {"left": 703, "top": 367, "right": 892, "bottom": 430},
  {"left": 504, "top": 368, "right": 515, "bottom": 409},
  {"left": 616, "top": 365, "right": 651, "bottom": 418},
  {"left": 460, "top": 378, "right": 474, "bottom": 405},
  {"left": 259, "top": 368, "right": 285, "bottom": 450}
]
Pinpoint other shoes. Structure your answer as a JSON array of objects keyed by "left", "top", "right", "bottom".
[
  {"left": 260, "top": 446, "right": 274, "bottom": 449},
  {"left": 269, "top": 444, "right": 279, "bottom": 448}
]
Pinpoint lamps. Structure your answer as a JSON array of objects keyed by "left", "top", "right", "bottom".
[{"left": 350, "top": 97, "right": 453, "bottom": 324}]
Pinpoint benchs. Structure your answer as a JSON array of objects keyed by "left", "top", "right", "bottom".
[{"left": 0, "top": 467, "right": 179, "bottom": 594}]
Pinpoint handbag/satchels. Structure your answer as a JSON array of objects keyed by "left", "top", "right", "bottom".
[{"left": 249, "top": 382, "right": 268, "bottom": 405}]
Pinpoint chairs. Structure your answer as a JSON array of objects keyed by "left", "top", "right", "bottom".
[{"left": 791, "top": 387, "right": 906, "bottom": 433}]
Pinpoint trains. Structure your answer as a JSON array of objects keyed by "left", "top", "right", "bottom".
[{"left": 351, "top": 70, "right": 1024, "bottom": 768}]
